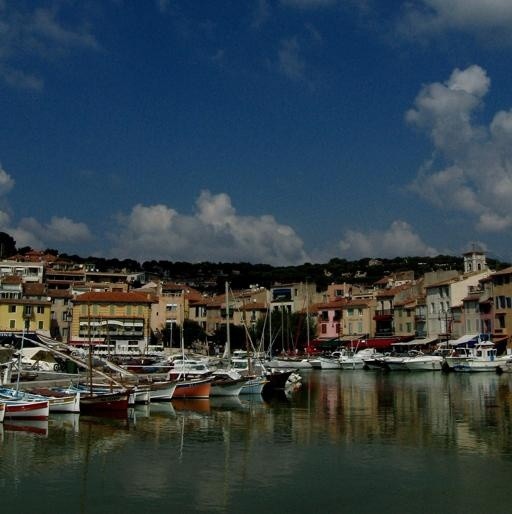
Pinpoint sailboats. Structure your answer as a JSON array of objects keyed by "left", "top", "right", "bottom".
[
  {"left": 3, "top": 419, "right": 52, "bottom": 440},
  {"left": 0, "top": 274, "right": 308, "bottom": 415}
]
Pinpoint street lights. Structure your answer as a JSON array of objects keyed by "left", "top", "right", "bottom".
[{"left": 438, "top": 306, "right": 456, "bottom": 348}]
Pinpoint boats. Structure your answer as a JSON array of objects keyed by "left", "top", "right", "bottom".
[
  {"left": 0, "top": 387, "right": 82, "bottom": 413},
  {"left": 0, "top": 319, "right": 50, "bottom": 418},
  {"left": 308, "top": 336, "right": 512, "bottom": 373}
]
